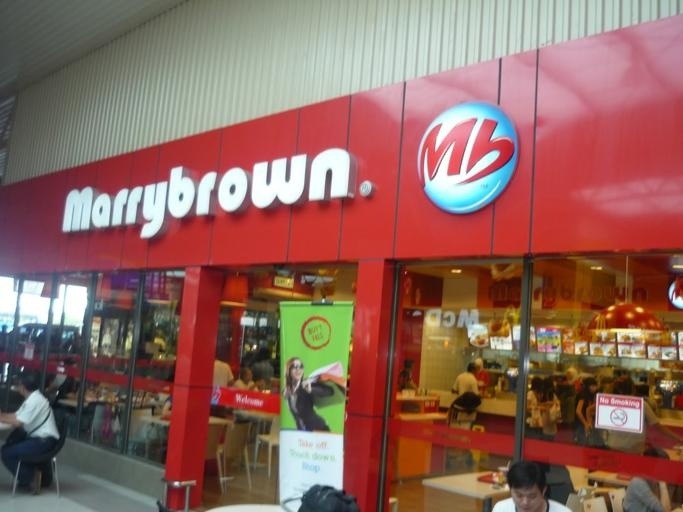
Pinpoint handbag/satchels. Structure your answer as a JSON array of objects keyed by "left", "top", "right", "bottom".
[
  {"left": 6, "top": 426, "right": 26, "bottom": 444},
  {"left": 309, "top": 361, "right": 347, "bottom": 409},
  {"left": 298, "top": 485, "right": 359, "bottom": 512}
]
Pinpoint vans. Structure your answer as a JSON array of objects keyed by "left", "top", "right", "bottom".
[{"left": 7, "top": 323, "right": 81, "bottom": 354}]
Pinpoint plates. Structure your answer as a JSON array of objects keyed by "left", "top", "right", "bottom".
[{"left": 469, "top": 328, "right": 489, "bottom": 348}]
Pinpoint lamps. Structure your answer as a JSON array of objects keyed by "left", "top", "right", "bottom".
[
  {"left": 586, "top": 256, "right": 668, "bottom": 333},
  {"left": 39, "top": 275, "right": 61, "bottom": 299},
  {"left": 94, "top": 267, "right": 247, "bottom": 307}
]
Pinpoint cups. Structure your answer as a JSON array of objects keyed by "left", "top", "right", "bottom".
[
  {"left": 402, "top": 389, "right": 416, "bottom": 398},
  {"left": 498, "top": 467, "right": 510, "bottom": 483}
]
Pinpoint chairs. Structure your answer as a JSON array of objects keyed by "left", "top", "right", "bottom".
[
  {"left": 0, "top": 386, "right": 279, "bottom": 500},
  {"left": 564, "top": 464, "right": 630, "bottom": 512}
]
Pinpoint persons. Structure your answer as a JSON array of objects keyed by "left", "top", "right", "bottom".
[
  {"left": 621, "top": 446, "right": 682, "bottom": 511},
  {"left": 525, "top": 355, "right": 683, "bottom": 455},
  {"left": 209, "top": 346, "right": 234, "bottom": 418},
  {"left": 249, "top": 345, "right": 274, "bottom": 383},
  {"left": 281, "top": 356, "right": 335, "bottom": 433},
  {"left": 450, "top": 362, "right": 493, "bottom": 399},
  {"left": 489, "top": 459, "right": 573, "bottom": 512},
  {"left": 0, "top": 370, "right": 60, "bottom": 496},
  {"left": 231, "top": 365, "right": 265, "bottom": 445},
  {"left": 402, "top": 357, "right": 416, "bottom": 389},
  {"left": 398, "top": 371, "right": 413, "bottom": 390},
  {"left": 471, "top": 356, "right": 490, "bottom": 395},
  {"left": 523, "top": 431, "right": 574, "bottom": 504}
]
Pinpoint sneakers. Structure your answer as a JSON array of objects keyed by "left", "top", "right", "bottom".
[{"left": 31, "top": 461, "right": 54, "bottom": 494}]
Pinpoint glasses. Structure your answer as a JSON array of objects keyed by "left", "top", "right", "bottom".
[{"left": 290, "top": 364, "right": 303, "bottom": 369}]
